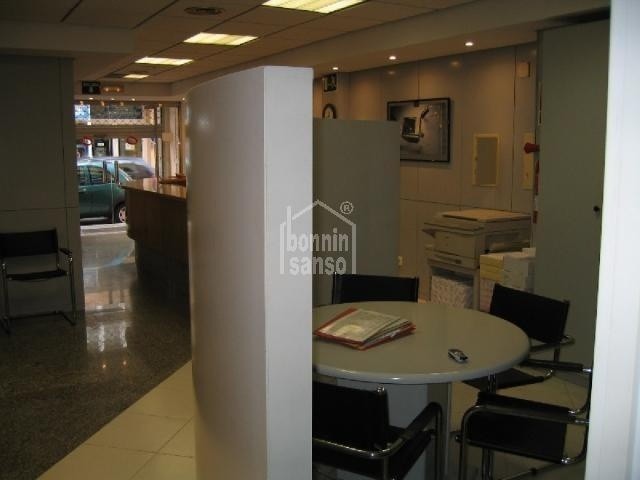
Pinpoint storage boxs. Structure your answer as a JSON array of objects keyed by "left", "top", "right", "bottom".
[{"left": 477, "top": 243, "right": 537, "bottom": 312}]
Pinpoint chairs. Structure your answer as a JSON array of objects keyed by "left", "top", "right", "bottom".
[
  {"left": 454, "top": 282, "right": 576, "bottom": 443},
  {"left": 327, "top": 270, "right": 422, "bottom": 303},
  {"left": 313, "top": 370, "right": 448, "bottom": 480},
  {"left": 452, "top": 349, "right": 595, "bottom": 480},
  {"left": 0, "top": 224, "right": 81, "bottom": 333}
]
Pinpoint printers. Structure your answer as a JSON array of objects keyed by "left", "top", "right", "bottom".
[{"left": 434, "top": 209, "right": 530, "bottom": 259}]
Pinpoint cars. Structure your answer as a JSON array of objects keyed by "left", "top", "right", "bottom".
[
  {"left": 86, "top": 156, "right": 155, "bottom": 179},
  {"left": 77, "top": 160, "right": 135, "bottom": 223}
]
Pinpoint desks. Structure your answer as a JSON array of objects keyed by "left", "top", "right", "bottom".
[{"left": 312, "top": 299, "right": 534, "bottom": 479}]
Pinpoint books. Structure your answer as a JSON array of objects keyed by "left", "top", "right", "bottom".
[{"left": 313, "top": 307, "right": 416, "bottom": 352}]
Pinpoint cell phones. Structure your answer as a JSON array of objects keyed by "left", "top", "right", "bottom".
[{"left": 448, "top": 348, "right": 469, "bottom": 362}]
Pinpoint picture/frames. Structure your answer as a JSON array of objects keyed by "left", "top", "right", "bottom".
[
  {"left": 322, "top": 72, "right": 339, "bottom": 97},
  {"left": 386, "top": 96, "right": 453, "bottom": 166}
]
